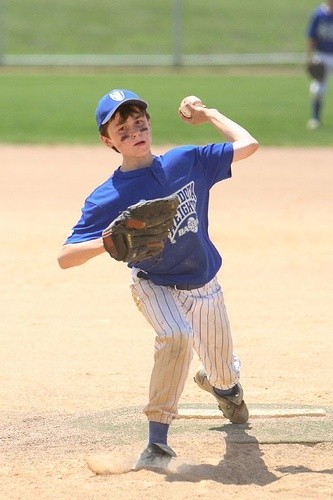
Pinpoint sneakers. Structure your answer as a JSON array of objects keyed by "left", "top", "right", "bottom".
[
  {"left": 193, "top": 368, "right": 249, "bottom": 424},
  {"left": 145, "top": 442, "right": 176, "bottom": 459}
]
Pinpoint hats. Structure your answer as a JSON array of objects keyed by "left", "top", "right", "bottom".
[{"left": 96, "top": 89, "right": 148, "bottom": 127}]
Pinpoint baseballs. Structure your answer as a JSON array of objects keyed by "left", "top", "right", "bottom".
[{"left": 180, "top": 96, "right": 203, "bottom": 118}]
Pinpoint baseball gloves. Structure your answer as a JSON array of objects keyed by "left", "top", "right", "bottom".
[{"left": 101, "top": 195, "right": 179, "bottom": 263}]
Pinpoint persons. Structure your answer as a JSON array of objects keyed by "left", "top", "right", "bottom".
[
  {"left": 57, "top": 89, "right": 259, "bottom": 474},
  {"left": 307, "top": 0, "right": 333, "bottom": 132}
]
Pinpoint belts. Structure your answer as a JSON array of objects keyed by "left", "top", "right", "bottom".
[{"left": 137, "top": 271, "right": 203, "bottom": 291}]
